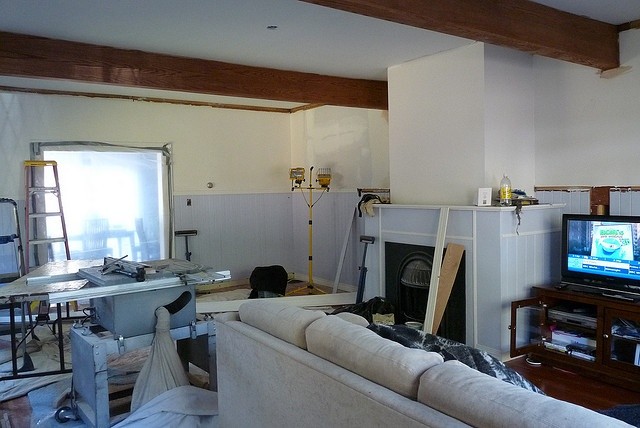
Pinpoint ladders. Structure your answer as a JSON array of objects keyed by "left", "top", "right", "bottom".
[
  {"left": 24, "top": 160, "right": 71, "bottom": 275},
  {"left": 0, "top": 198, "right": 35, "bottom": 340}
]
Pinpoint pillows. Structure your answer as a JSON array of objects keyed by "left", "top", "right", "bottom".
[{"left": 367, "top": 324, "right": 548, "bottom": 395}]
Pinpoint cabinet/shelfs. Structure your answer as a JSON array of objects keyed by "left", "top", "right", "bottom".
[{"left": 509, "top": 282, "right": 640, "bottom": 393}]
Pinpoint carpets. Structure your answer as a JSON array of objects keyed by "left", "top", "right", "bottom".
[{"left": 596, "top": 403, "right": 640, "bottom": 427}]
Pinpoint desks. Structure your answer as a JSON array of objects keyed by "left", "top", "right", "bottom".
[{"left": 0, "top": 260, "right": 231, "bottom": 380}]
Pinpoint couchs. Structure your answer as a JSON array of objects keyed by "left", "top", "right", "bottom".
[{"left": 214, "top": 299, "right": 637, "bottom": 428}]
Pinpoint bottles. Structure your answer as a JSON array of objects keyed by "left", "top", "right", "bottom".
[{"left": 500, "top": 173, "right": 512, "bottom": 207}]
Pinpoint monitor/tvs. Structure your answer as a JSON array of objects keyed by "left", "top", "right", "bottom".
[{"left": 560, "top": 214, "right": 640, "bottom": 302}]
